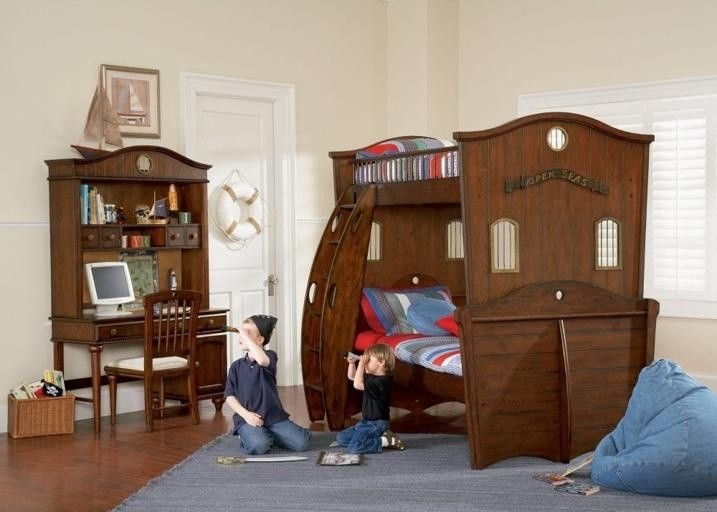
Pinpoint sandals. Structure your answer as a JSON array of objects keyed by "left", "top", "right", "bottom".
[{"left": 381, "top": 430, "right": 405, "bottom": 451}]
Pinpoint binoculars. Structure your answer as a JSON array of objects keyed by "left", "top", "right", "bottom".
[
  {"left": 221, "top": 325, "right": 239, "bottom": 332},
  {"left": 343, "top": 351, "right": 361, "bottom": 360}
]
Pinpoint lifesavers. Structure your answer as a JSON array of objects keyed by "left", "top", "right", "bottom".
[{"left": 217, "top": 182, "right": 264, "bottom": 243}]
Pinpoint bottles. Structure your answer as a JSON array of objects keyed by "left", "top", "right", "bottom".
[
  {"left": 168, "top": 270, "right": 178, "bottom": 307},
  {"left": 152, "top": 279, "right": 161, "bottom": 316},
  {"left": 168, "top": 183, "right": 179, "bottom": 211}
]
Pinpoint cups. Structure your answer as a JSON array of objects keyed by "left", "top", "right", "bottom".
[
  {"left": 179, "top": 212, "right": 192, "bottom": 224},
  {"left": 105, "top": 204, "right": 116, "bottom": 224},
  {"left": 121, "top": 234, "right": 128, "bottom": 248}
]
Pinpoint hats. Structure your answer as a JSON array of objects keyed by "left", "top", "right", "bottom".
[{"left": 250, "top": 315, "right": 277, "bottom": 346}]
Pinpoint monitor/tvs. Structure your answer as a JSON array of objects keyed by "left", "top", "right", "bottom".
[{"left": 84, "top": 262, "right": 136, "bottom": 318}]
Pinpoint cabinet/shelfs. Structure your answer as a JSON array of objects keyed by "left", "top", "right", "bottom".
[{"left": 43, "top": 146, "right": 231, "bottom": 433}]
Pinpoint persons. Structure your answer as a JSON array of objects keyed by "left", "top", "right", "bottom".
[
  {"left": 222, "top": 313, "right": 314, "bottom": 456},
  {"left": 334, "top": 342, "right": 406, "bottom": 455}
]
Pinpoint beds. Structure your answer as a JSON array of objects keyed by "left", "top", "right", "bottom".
[{"left": 300, "top": 112, "right": 661, "bottom": 470}]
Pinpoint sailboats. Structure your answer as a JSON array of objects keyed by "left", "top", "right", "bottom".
[{"left": 115, "top": 82, "right": 149, "bottom": 127}]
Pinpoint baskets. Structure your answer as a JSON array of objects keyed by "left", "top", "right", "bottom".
[{"left": 8, "top": 394, "right": 76, "bottom": 440}]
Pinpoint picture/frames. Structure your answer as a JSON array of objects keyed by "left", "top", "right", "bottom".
[
  {"left": 100, "top": 64, "right": 160, "bottom": 139},
  {"left": 120, "top": 249, "right": 160, "bottom": 312}
]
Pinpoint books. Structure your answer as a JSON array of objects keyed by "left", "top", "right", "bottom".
[
  {"left": 531, "top": 471, "right": 576, "bottom": 487},
  {"left": 560, "top": 450, "right": 594, "bottom": 478},
  {"left": 9, "top": 369, "right": 66, "bottom": 402},
  {"left": 78, "top": 183, "right": 109, "bottom": 225},
  {"left": 552, "top": 481, "right": 601, "bottom": 497}
]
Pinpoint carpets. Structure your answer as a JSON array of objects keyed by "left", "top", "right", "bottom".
[{"left": 112, "top": 430, "right": 717, "bottom": 512}]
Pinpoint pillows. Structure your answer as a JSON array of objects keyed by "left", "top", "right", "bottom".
[
  {"left": 355, "top": 138, "right": 455, "bottom": 163},
  {"left": 357, "top": 283, "right": 460, "bottom": 339}
]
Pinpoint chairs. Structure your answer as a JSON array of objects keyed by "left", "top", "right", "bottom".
[
  {"left": 104, "top": 290, "right": 202, "bottom": 433},
  {"left": 591, "top": 358, "right": 716, "bottom": 496}
]
